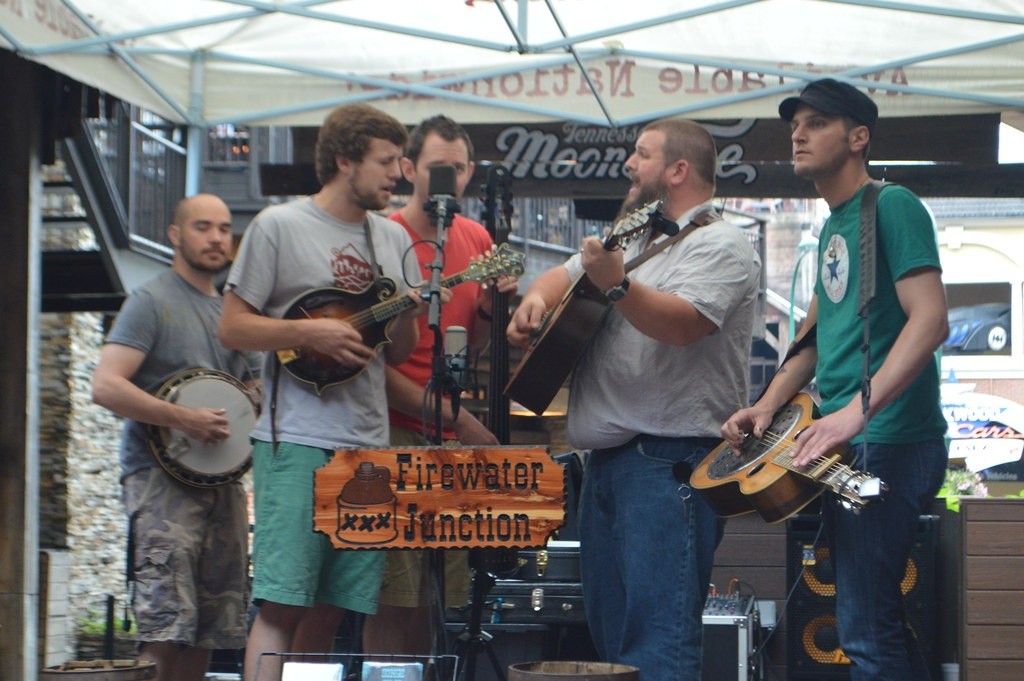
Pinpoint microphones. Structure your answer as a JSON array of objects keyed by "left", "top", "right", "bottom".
[{"left": 422, "top": 165, "right": 460, "bottom": 227}]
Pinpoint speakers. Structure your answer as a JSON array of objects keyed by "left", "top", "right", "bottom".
[{"left": 787, "top": 514, "right": 942, "bottom": 681}]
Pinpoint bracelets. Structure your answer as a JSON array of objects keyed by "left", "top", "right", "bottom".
[{"left": 477, "top": 304, "right": 492, "bottom": 321}]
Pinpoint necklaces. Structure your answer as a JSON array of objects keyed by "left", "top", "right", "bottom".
[{"left": 828, "top": 175, "right": 872, "bottom": 259}]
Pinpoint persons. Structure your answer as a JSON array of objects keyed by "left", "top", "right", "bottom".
[
  {"left": 362, "top": 115, "right": 518, "bottom": 676},
  {"left": 506, "top": 119, "right": 762, "bottom": 681},
  {"left": 217, "top": 105, "right": 454, "bottom": 681},
  {"left": 93, "top": 194, "right": 265, "bottom": 681},
  {"left": 721, "top": 78, "right": 949, "bottom": 681}
]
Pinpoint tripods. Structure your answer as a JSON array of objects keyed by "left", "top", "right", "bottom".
[{"left": 448, "top": 546, "right": 506, "bottom": 681}]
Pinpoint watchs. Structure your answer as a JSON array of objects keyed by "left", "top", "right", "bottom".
[{"left": 599, "top": 276, "right": 629, "bottom": 303}]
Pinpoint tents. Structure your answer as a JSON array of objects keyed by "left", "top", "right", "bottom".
[{"left": 0, "top": 0, "right": 1024, "bottom": 681}]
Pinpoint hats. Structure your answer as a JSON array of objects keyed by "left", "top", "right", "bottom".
[{"left": 779, "top": 78, "right": 878, "bottom": 133}]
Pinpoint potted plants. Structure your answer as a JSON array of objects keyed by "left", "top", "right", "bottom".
[{"left": 78, "top": 610, "right": 139, "bottom": 661}]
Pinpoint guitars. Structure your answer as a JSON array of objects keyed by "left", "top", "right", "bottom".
[
  {"left": 689, "top": 391, "right": 891, "bottom": 526},
  {"left": 146, "top": 368, "right": 263, "bottom": 489},
  {"left": 502, "top": 199, "right": 681, "bottom": 418},
  {"left": 274, "top": 241, "right": 526, "bottom": 398}
]
